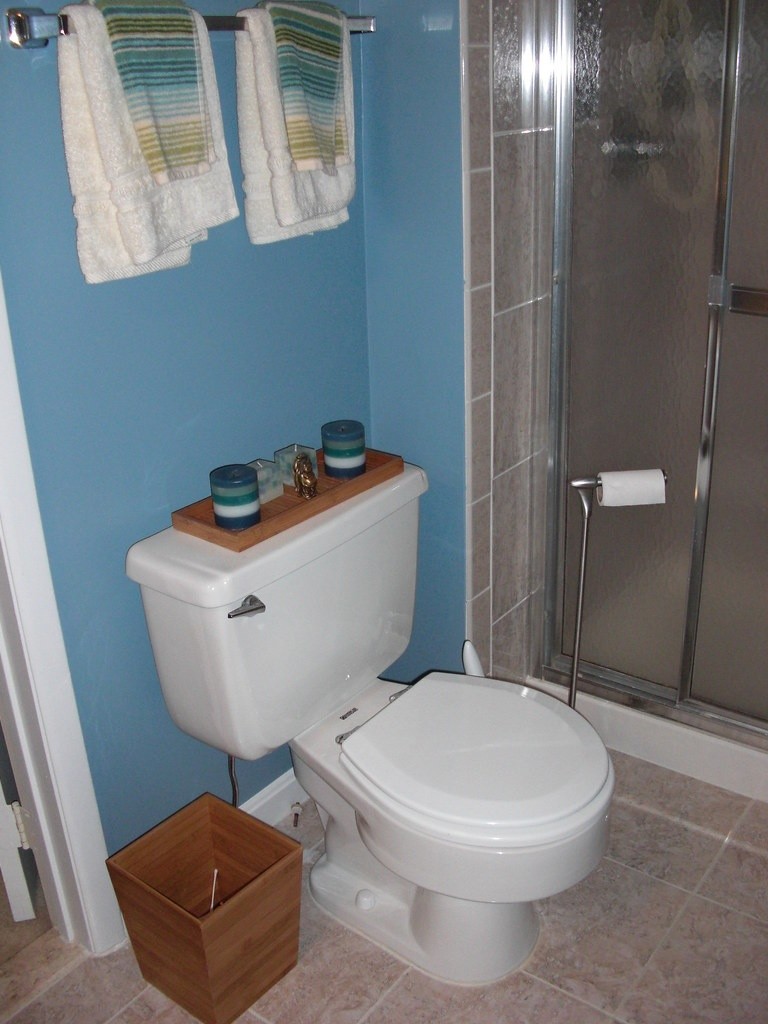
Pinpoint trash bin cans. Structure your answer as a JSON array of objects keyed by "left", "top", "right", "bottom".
[{"left": 102, "top": 788, "right": 307, "bottom": 1024}]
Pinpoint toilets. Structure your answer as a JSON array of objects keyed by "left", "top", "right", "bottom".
[{"left": 128, "top": 461, "right": 614, "bottom": 985}]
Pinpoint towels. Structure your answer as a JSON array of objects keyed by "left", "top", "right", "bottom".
[
  {"left": 235, "top": 4, "right": 355, "bottom": 245},
  {"left": 52, "top": 1, "right": 241, "bottom": 287}
]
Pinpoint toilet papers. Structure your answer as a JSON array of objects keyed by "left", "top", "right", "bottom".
[{"left": 597, "top": 469, "right": 669, "bottom": 508}]
